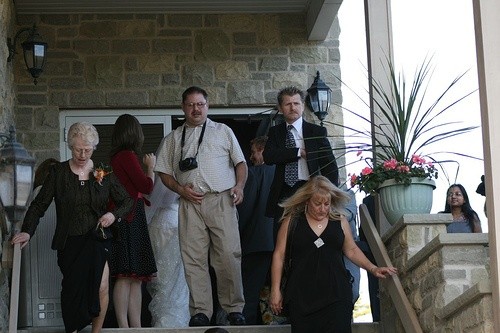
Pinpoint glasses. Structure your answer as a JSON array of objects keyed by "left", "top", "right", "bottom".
[
  {"left": 71, "top": 146, "right": 93, "bottom": 155},
  {"left": 182, "top": 99, "right": 207, "bottom": 108}
]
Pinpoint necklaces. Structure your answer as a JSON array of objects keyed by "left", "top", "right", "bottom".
[
  {"left": 318, "top": 225, "right": 322, "bottom": 228},
  {"left": 80, "top": 172, "right": 84, "bottom": 176}
]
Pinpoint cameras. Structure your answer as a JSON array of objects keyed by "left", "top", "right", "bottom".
[{"left": 179, "top": 157, "right": 198, "bottom": 171}]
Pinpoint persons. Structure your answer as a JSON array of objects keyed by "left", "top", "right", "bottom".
[
  {"left": 438, "top": 184, "right": 482, "bottom": 233},
  {"left": 268, "top": 175, "right": 397, "bottom": 333},
  {"left": 106, "top": 114, "right": 156, "bottom": 328},
  {"left": 33, "top": 158, "right": 59, "bottom": 198},
  {"left": 338, "top": 189, "right": 361, "bottom": 304},
  {"left": 11, "top": 120, "right": 134, "bottom": 333},
  {"left": 237, "top": 135, "right": 276, "bottom": 325},
  {"left": 262, "top": 87, "right": 339, "bottom": 246},
  {"left": 143, "top": 176, "right": 191, "bottom": 328},
  {"left": 153, "top": 86, "right": 249, "bottom": 328}
]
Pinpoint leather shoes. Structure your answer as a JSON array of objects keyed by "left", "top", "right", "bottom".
[
  {"left": 189, "top": 313, "right": 209, "bottom": 327},
  {"left": 226, "top": 312, "right": 246, "bottom": 325}
]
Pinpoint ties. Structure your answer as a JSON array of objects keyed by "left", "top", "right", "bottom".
[{"left": 285, "top": 125, "right": 298, "bottom": 188}]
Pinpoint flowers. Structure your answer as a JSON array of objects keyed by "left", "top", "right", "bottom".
[
  {"left": 296, "top": 47, "right": 485, "bottom": 201},
  {"left": 92, "top": 163, "right": 113, "bottom": 187}
]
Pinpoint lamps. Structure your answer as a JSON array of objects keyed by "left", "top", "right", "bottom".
[
  {"left": 0, "top": 126, "right": 37, "bottom": 235},
  {"left": 304, "top": 69, "right": 332, "bottom": 127},
  {"left": 5, "top": 23, "right": 48, "bottom": 85}
]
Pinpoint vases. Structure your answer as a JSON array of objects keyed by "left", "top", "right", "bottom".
[{"left": 377, "top": 177, "right": 437, "bottom": 225}]
